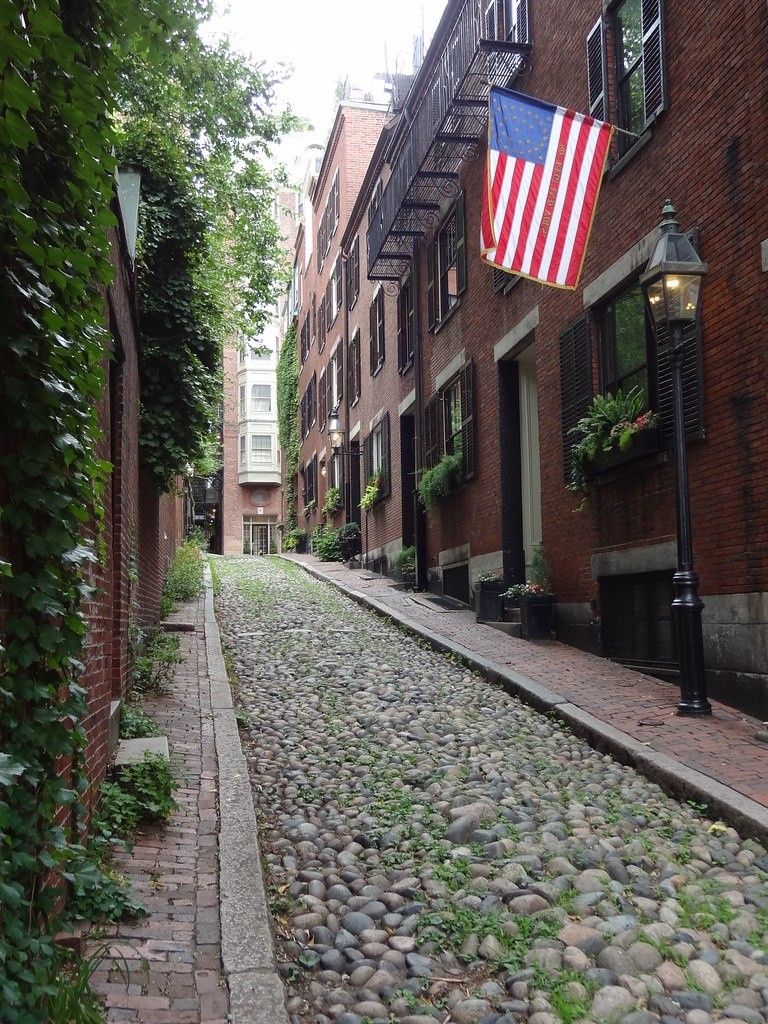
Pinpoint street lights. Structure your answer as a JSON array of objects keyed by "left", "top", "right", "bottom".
[{"left": 640, "top": 195, "right": 710, "bottom": 716}]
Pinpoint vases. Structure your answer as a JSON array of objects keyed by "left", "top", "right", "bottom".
[
  {"left": 518, "top": 594, "right": 555, "bottom": 643},
  {"left": 474, "top": 581, "right": 503, "bottom": 622},
  {"left": 336, "top": 538, "right": 359, "bottom": 563}
]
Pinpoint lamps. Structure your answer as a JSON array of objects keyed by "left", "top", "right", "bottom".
[{"left": 327, "top": 407, "right": 366, "bottom": 456}]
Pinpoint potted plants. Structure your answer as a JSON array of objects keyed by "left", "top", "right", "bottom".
[{"left": 291, "top": 528, "right": 307, "bottom": 554}]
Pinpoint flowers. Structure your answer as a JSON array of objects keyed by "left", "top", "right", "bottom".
[
  {"left": 477, "top": 571, "right": 504, "bottom": 582},
  {"left": 336, "top": 522, "right": 360, "bottom": 542},
  {"left": 498, "top": 581, "right": 554, "bottom": 597},
  {"left": 608, "top": 410, "right": 661, "bottom": 437}
]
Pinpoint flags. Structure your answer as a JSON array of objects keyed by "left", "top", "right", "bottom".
[{"left": 481, "top": 86, "right": 613, "bottom": 291}]
